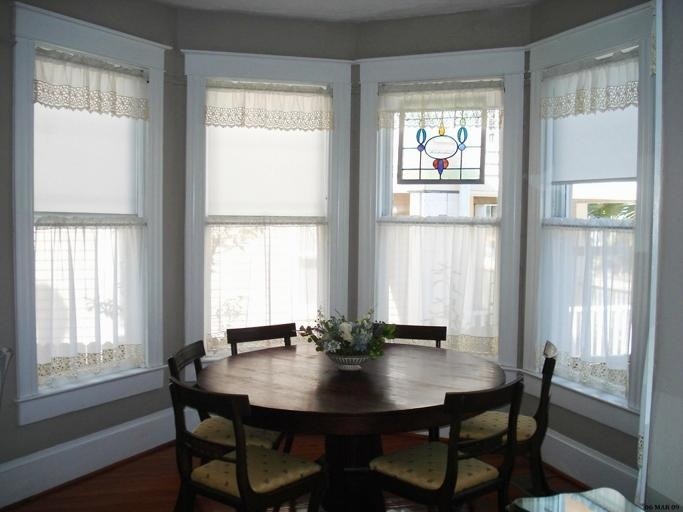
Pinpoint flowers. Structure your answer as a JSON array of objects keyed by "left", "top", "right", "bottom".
[{"left": 292, "top": 305, "right": 397, "bottom": 360}]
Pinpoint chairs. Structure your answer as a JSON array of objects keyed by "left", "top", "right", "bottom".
[
  {"left": 368, "top": 375, "right": 524, "bottom": 512},
  {"left": 226, "top": 323, "right": 297, "bottom": 356},
  {"left": 381, "top": 325, "right": 447, "bottom": 442},
  {"left": 167, "top": 339, "right": 296, "bottom": 466},
  {"left": 167, "top": 376, "right": 328, "bottom": 512},
  {"left": 459, "top": 340, "right": 559, "bottom": 497}
]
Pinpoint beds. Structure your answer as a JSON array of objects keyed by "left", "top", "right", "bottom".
[{"left": 505, "top": 486, "right": 647, "bottom": 512}]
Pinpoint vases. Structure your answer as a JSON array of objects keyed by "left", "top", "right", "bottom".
[{"left": 325, "top": 350, "right": 370, "bottom": 371}]
